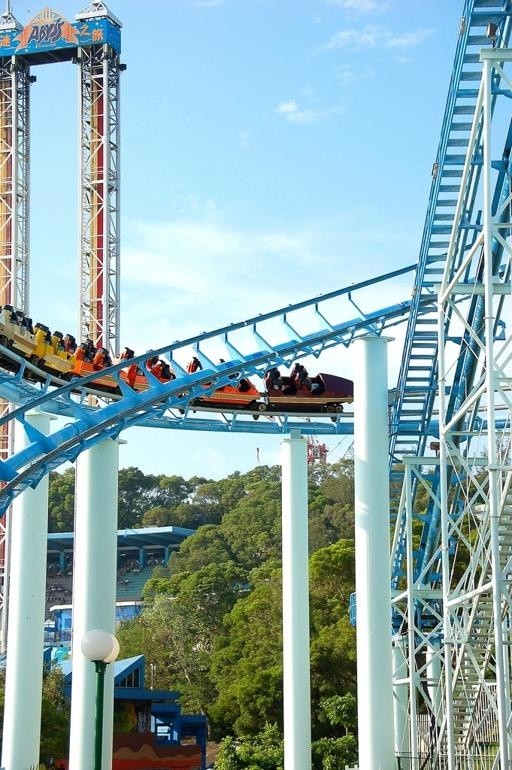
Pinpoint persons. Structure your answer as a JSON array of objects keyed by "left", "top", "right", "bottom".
[
  {"left": 185, "top": 356, "right": 212, "bottom": 386},
  {"left": 264, "top": 362, "right": 311, "bottom": 397},
  {"left": 2, "top": 305, "right": 176, "bottom": 380},
  {"left": 218, "top": 358, "right": 249, "bottom": 391},
  {"left": 45, "top": 556, "right": 159, "bottom": 602}
]
